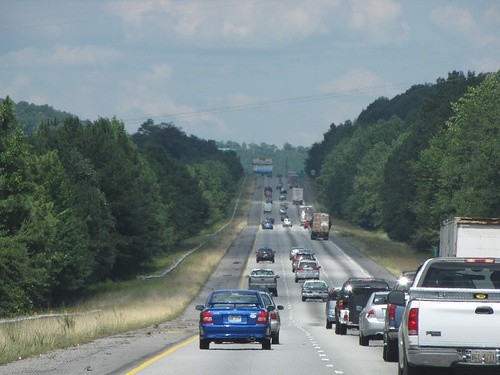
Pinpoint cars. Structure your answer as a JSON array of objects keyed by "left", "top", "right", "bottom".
[
  {"left": 355, "top": 291, "right": 393, "bottom": 346},
  {"left": 258, "top": 291, "right": 284, "bottom": 344},
  {"left": 325, "top": 287, "right": 343, "bottom": 329},
  {"left": 383, "top": 270, "right": 420, "bottom": 363},
  {"left": 255, "top": 247, "right": 275, "bottom": 263},
  {"left": 261, "top": 185, "right": 292, "bottom": 230},
  {"left": 301, "top": 280, "right": 329, "bottom": 302},
  {"left": 289, "top": 247, "right": 321, "bottom": 282},
  {"left": 195, "top": 289, "right": 275, "bottom": 350}
]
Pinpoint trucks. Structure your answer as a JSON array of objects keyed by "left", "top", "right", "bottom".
[
  {"left": 432, "top": 216, "right": 500, "bottom": 257},
  {"left": 298, "top": 205, "right": 315, "bottom": 226},
  {"left": 291, "top": 187, "right": 304, "bottom": 204},
  {"left": 311, "top": 212, "right": 330, "bottom": 240}
]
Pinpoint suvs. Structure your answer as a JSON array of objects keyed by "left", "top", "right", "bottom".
[{"left": 335, "top": 277, "right": 391, "bottom": 335}]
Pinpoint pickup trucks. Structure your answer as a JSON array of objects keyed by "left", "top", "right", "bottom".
[
  {"left": 245, "top": 269, "right": 280, "bottom": 296},
  {"left": 387, "top": 257, "right": 500, "bottom": 375}
]
